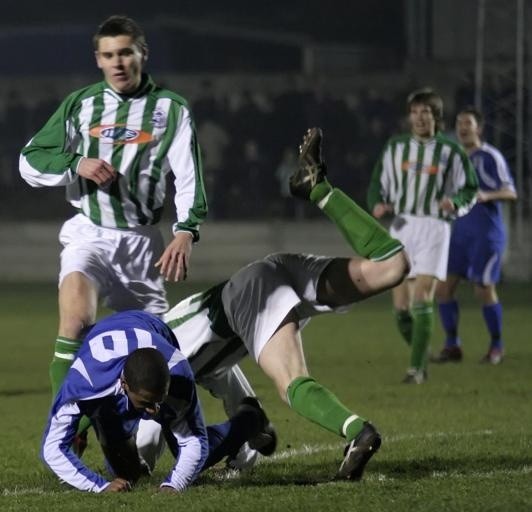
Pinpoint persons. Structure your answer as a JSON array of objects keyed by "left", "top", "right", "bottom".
[
  {"left": 365, "top": 87, "right": 478, "bottom": 385},
  {"left": 77, "top": 127, "right": 411, "bottom": 480},
  {"left": 429, "top": 107, "right": 519, "bottom": 365},
  {"left": 40, "top": 307, "right": 272, "bottom": 494},
  {"left": 19, "top": 15, "right": 210, "bottom": 456}
]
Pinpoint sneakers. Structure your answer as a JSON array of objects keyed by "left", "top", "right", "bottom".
[
  {"left": 73, "top": 431, "right": 87, "bottom": 458},
  {"left": 400, "top": 368, "right": 429, "bottom": 384},
  {"left": 288, "top": 125, "right": 327, "bottom": 199},
  {"left": 240, "top": 397, "right": 277, "bottom": 457},
  {"left": 430, "top": 345, "right": 463, "bottom": 363},
  {"left": 330, "top": 419, "right": 381, "bottom": 484},
  {"left": 481, "top": 348, "right": 503, "bottom": 365}
]
